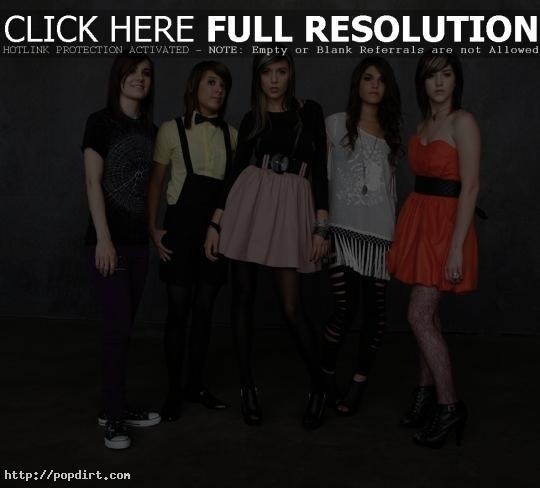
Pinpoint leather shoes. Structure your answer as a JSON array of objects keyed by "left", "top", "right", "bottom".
[
  {"left": 242, "top": 382, "right": 261, "bottom": 424},
  {"left": 303, "top": 389, "right": 327, "bottom": 430},
  {"left": 187, "top": 383, "right": 227, "bottom": 409},
  {"left": 336, "top": 369, "right": 367, "bottom": 416},
  {"left": 166, "top": 394, "right": 182, "bottom": 421}
]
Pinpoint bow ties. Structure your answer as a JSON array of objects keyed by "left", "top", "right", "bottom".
[{"left": 195, "top": 113, "right": 220, "bottom": 127}]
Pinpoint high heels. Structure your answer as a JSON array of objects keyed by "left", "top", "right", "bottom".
[
  {"left": 400, "top": 385, "right": 435, "bottom": 427},
  {"left": 413, "top": 403, "right": 467, "bottom": 449}
]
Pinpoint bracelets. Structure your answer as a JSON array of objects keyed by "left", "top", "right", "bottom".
[
  {"left": 313, "top": 218, "right": 330, "bottom": 241},
  {"left": 210, "top": 219, "right": 220, "bottom": 233}
]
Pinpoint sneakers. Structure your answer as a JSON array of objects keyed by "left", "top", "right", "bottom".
[
  {"left": 99, "top": 406, "right": 162, "bottom": 428},
  {"left": 105, "top": 417, "right": 131, "bottom": 449}
]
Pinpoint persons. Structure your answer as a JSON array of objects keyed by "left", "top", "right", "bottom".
[
  {"left": 146, "top": 61, "right": 241, "bottom": 420},
  {"left": 80, "top": 51, "right": 159, "bottom": 450},
  {"left": 385, "top": 47, "right": 489, "bottom": 447},
  {"left": 203, "top": 40, "right": 329, "bottom": 431},
  {"left": 324, "top": 54, "right": 402, "bottom": 416}
]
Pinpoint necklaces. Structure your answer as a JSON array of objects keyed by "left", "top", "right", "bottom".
[{"left": 359, "top": 126, "right": 380, "bottom": 196}]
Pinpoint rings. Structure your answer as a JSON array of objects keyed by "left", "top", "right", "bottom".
[{"left": 451, "top": 272, "right": 459, "bottom": 280}]
